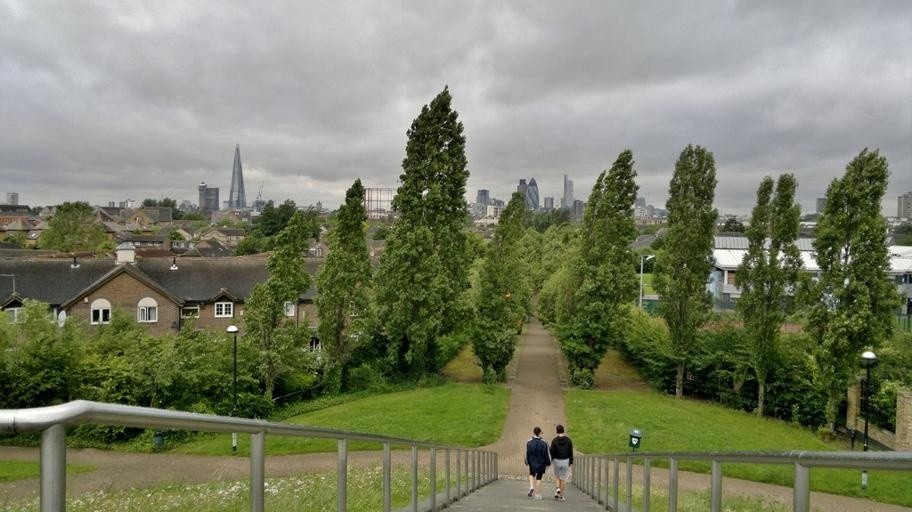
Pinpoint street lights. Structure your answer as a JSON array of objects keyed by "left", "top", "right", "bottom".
[
  {"left": 862, "top": 351, "right": 877, "bottom": 487},
  {"left": 639, "top": 253, "right": 656, "bottom": 309},
  {"left": 226, "top": 325, "right": 240, "bottom": 452}
]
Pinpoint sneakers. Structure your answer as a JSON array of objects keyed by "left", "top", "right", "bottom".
[
  {"left": 555, "top": 488, "right": 565, "bottom": 501},
  {"left": 528, "top": 488, "right": 534, "bottom": 498},
  {"left": 534, "top": 494, "right": 542, "bottom": 499}
]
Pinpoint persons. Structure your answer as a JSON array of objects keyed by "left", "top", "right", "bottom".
[
  {"left": 525, "top": 427, "right": 552, "bottom": 500},
  {"left": 549, "top": 425, "right": 574, "bottom": 501}
]
